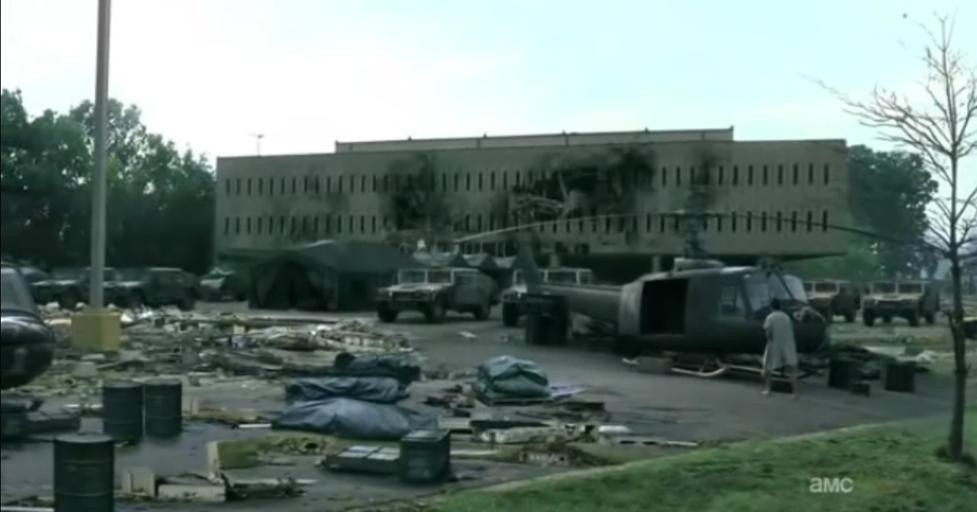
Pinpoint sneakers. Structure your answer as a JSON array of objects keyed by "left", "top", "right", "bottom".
[{"left": 761, "top": 389, "right": 801, "bottom": 401}]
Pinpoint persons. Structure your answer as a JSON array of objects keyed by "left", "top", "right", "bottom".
[{"left": 761, "top": 297, "right": 801, "bottom": 400}]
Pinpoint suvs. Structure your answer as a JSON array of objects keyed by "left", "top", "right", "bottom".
[
  {"left": 803, "top": 279, "right": 860, "bottom": 323},
  {"left": 30, "top": 267, "right": 198, "bottom": 310},
  {"left": 862, "top": 281, "right": 940, "bottom": 326},
  {"left": 500, "top": 267, "right": 592, "bottom": 327},
  {"left": 375, "top": 267, "right": 494, "bottom": 323}
]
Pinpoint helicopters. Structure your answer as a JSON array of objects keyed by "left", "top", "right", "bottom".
[{"left": 451, "top": 210, "right": 907, "bottom": 379}]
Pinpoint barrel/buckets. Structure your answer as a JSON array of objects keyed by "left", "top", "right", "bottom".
[
  {"left": 146, "top": 378, "right": 183, "bottom": 436},
  {"left": 886, "top": 363, "right": 913, "bottom": 391},
  {"left": 103, "top": 381, "right": 142, "bottom": 441},
  {"left": 54, "top": 434, "right": 114, "bottom": 512}
]
